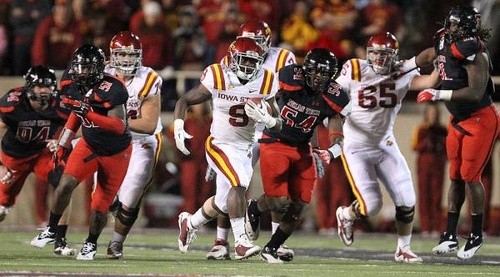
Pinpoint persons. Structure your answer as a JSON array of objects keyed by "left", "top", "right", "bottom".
[
  {"left": 173, "top": 36, "right": 280, "bottom": 260},
  {"left": 0, "top": 0, "right": 500, "bottom": 115},
  {"left": 30, "top": 44, "right": 132, "bottom": 260},
  {"left": 314, "top": 113, "right": 354, "bottom": 236},
  {"left": 205, "top": 18, "right": 297, "bottom": 260},
  {"left": 316, "top": 31, "right": 443, "bottom": 263},
  {"left": 166, "top": 103, "right": 213, "bottom": 230},
  {"left": 92, "top": 31, "right": 163, "bottom": 259},
  {"left": 394, "top": 5, "right": 500, "bottom": 260},
  {"left": 0, "top": 65, "right": 73, "bottom": 244},
  {"left": 244, "top": 48, "right": 350, "bottom": 263},
  {"left": 411, "top": 104, "right": 448, "bottom": 240}
]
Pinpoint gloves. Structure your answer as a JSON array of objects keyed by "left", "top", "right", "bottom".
[
  {"left": 52, "top": 147, "right": 67, "bottom": 173},
  {"left": 173, "top": 119, "right": 193, "bottom": 156},
  {"left": 60, "top": 99, "right": 91, "bottom": 117},
  {"left": 418, "top": 89, "right": 453, "bottom": 104},
  {"left": 245, "top": 98, "right": 275, "bottom": 129}
]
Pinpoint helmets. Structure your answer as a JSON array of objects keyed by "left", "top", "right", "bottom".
[
  {"left": 24, "top": 65, "right": 57, "bottom": 102},
  {"left": 227, "top": 37, "right": 261, "bottom": 82},
  {"left": 368, "top": 31, "right": 398, "bottom": 75},
  {"left": 444, "top": 5, "right": 479, "bottom": 41},
  {"left": 238, "top": 19, "right": 271, "bottom": 54},
  {"left": 303, "top": 48, "right": 338, "bottom": 94},
  {"left": 71, "top": 46, "right": 105, "bottom": 93},
  {"left": 110, "top": 32, "right": 141, "bottom": 73}
]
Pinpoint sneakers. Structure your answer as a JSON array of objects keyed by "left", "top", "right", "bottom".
[
  {"left": 276, "top": 244, "right": 294, "bottom": 261},
  {"left": 457, "top": 234, "right": 483, "bottom": 259},
  {"left": 261, "top": 247, "right": 284, "bottom": 263},
  {"left": 336, "top": 207, "right": 354, "bottom": 246},
  {"left": 433, "top": 233, "right": 458, "bottom": 254},
  {"left": 76, "top": 241, "right": 98, "bottom": 260},
  {"left": 178, "top": 212, "right": 198, "bottom": 255},
  {"left": 235, "top": 235, "right": 262, "bottom": 259},
  {"left": 52, "top": 240, "right": 76, "bottom": 256},
  {"left": 244, "top": 199, "right": 261, "bottom": 239},
  {"left": 30, "top": 224, "right": 56, "bottom": 247},
  {"left": 207, "top": 237, "right": 230, "bottom": 260},
  {"left": 395, "top": 246, "right": 424, "bottom": 265},
  {"left": 106, "top": 240, "right": 123, "bottom": 259}
]
